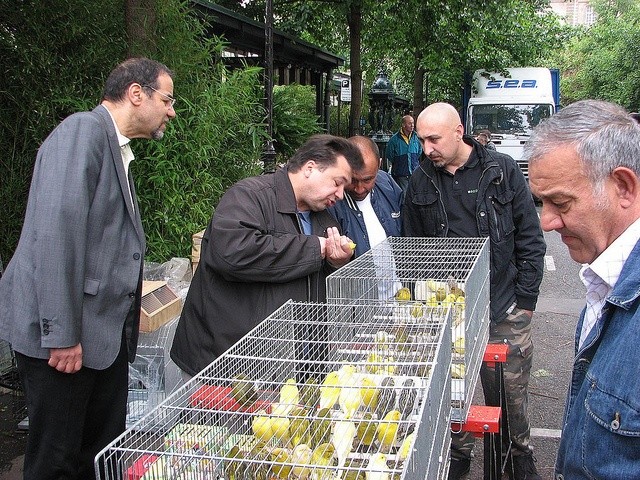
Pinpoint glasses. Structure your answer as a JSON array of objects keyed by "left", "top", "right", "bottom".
[{"left": 141, "top": 84, "right": 176, "bottom": 108}]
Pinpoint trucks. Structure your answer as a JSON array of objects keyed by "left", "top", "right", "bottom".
[{"left": 465, "top": 68, "right": 561, "bottom": 207}]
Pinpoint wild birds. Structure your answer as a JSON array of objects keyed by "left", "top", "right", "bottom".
[
  {"left": 224, "top": 365, "right": 417, "bottom": 480},
  {"left": 366, "top": 275, "right": 465, "bottom": 379}
]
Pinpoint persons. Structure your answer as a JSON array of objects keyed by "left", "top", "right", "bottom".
[
  {"left": 380, "top": 114, "right": 422, "bottom": 199},
  {"left": 522, "top": 99, "right": 640, "bottom": 480},
  {"left": 168, "top": 134, "right": 365, "bottom": 384},
  {"left": 481, "top": 129, "right": 496, "bottom": 151},
  {"left": 1, "top": 57, "right": 177, "bottom": 479},
  {"left": 475, "top": 132, "right": 494, "bottom": 151},
  {"left": 404, "top": 102, "right": 547, "bottom": 480},
  {"left": 327, "top": 136, "right": 404, "bottom": 303}
]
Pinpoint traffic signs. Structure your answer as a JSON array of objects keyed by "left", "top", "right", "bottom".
[{"left": 341, "top": 79, "right": 363, "bottom": 102}]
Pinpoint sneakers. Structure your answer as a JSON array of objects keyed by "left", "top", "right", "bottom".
[
  {"left": 503, "top": 453, "right": 543, "bottom": 480},
  {"left": 447, "top": 456, "right": 471, "bottom": 480}
]
